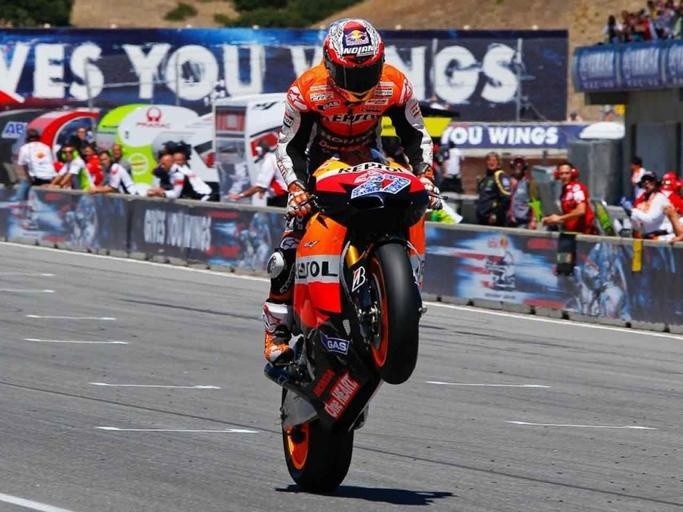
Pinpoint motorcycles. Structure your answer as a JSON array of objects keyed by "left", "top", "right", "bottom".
[{"left": 262, "top": 155, "right": 449, "bottom": 491}]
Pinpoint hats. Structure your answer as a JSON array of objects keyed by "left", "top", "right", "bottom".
[{"left": 657, "top": 170, "right": 683, "bottom": 194}]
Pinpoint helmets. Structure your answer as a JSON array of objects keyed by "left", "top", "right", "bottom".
[{"left": 318, "top": 14, "right": 389, "bottom": 106}]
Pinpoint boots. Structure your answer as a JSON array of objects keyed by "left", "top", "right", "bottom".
[{"left": 259, "top": 301, "right": 297, "bottom": 368}]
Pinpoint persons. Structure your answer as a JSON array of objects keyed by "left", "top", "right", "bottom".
[
  {"left": 2, "top": 127, "right": 290, "bottom": 205},
  {"left": 264, "top": 17, "right": 443, "bottom": 365},
  {"left": 421, "top": 138, "right": 683, "bottom": 245}
]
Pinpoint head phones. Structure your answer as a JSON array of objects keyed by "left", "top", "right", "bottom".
[
  {"left": 554, "top": 162, "right": 579, "bottom": 179},
  {"left": 509, "top": 154, "right": 529, "bottom": 170},
  {"left": 254, "top": 146, "right": 264, "bottom": 157},
  {"left": 61, "top": 144, "right": 78, "bottom": 161}
]
